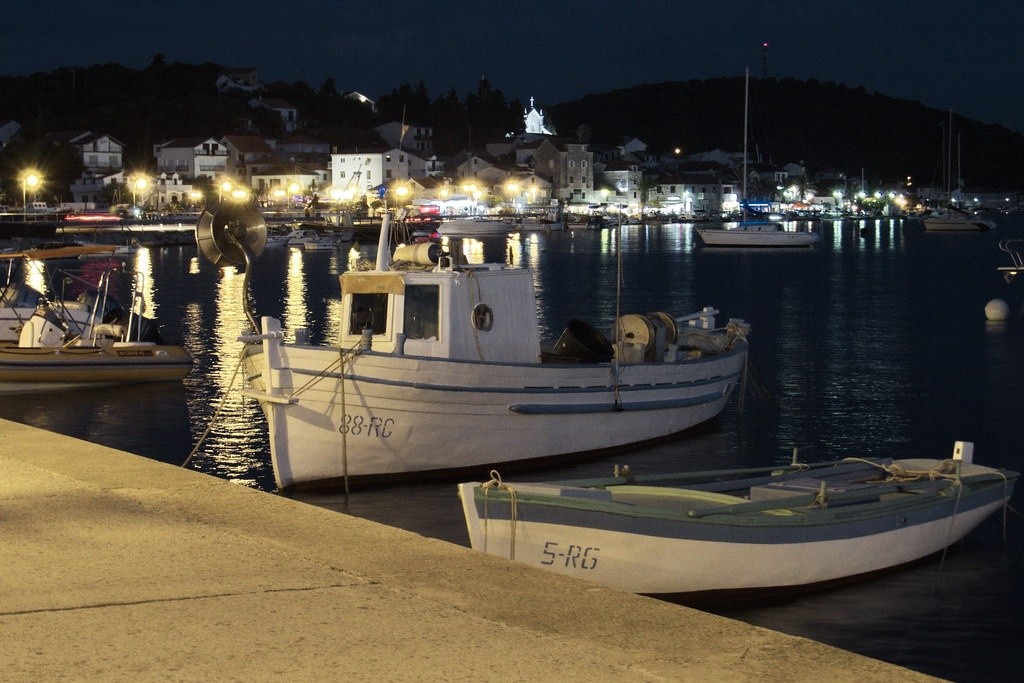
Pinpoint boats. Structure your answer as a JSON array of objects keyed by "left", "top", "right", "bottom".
[
  {"left": 455, "top": 439, "right": 1023, "bottom": 597},
  {"left": 0, "top": 201, "right": 752, "bottom": 488}
]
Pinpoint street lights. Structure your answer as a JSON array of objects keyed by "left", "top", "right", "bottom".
[
  {"left": 21, "top": 175, "right": 37, "bottom": 224},
  {"left": 133, "top": 181, "right": 147, "bottom": 209},
  {"left": 219, "top": 182, "right": 232, "bottom": 212}
]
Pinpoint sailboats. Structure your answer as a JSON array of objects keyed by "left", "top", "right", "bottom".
[
  {"left": 692, "top": 66, "right": 821, "bottom": 245},
  {"left": 923, "top": 108, "right": 993, "bottom": 230}
]
